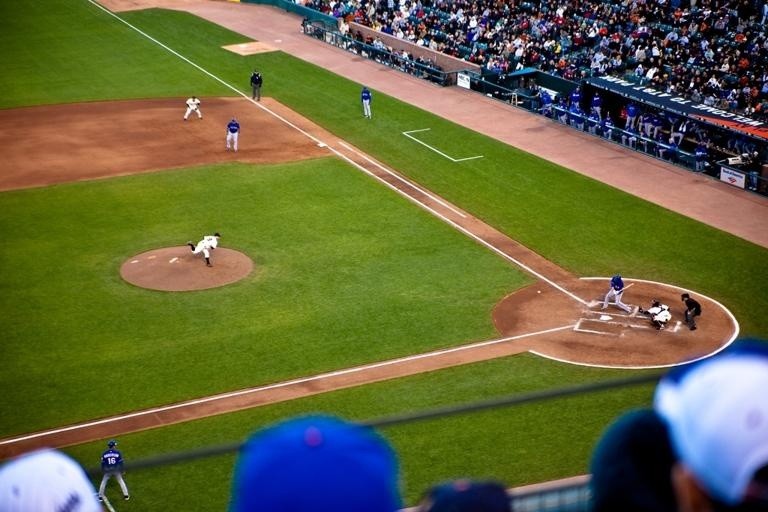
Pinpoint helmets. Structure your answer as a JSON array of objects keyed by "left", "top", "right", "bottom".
[{"left": 680, "top": 294, "right": 688, "bottom": 301}]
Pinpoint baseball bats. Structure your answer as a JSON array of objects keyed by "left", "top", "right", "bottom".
[{"left": 619, "top": 283, "right": 634, "bottom": 291}]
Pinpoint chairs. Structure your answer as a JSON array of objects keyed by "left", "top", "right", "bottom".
[
  {"left": 352, "top": 1, "right": 462, "bottom": 88},
  {"left": 462, "top": 0, "right": 767, "bottom": 182}
]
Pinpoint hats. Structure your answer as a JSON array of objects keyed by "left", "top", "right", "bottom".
[
  {"left": 107, "top": 441, "right": 117, "bottom": 446},
  {"left": 227, "top": 414, "right": 403, "bottom": 512},
  {"left": 421, "top": 480, "right": 512, "bottom": 512},
  {"left": 0, "top": 450, "right": 101, "bottom": 512},
  {"left": 651, "top": 338, "right": 767, "bottom": 506}
]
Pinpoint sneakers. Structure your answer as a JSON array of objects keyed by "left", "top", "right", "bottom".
[
  {"left": 124, "top": 495, "right": 130, "bottom": 501},
  {"left": 99, "top": 497, "right": 103, "bottom": 502}
]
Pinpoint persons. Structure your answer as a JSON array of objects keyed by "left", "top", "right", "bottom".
[
  {"left": 0, "top": 448, "right": 103, "bottom": 512},
  {"left": 681, "top": 294, "right": 701, "bottom": 330},
  {"left": 230, "top": 414, "right": 404, "bottom": 512},
  {"left": 297, "top": 2, "right": 766, "bottom": 124},
  {"left": 589, "top": 411, "right": 680, "bottom": 512},
  {"left": 225, "top": 116, "right": 240, "bottom": 153},
  {"left": 600, "top": 274, "right": 631, "bottom": 314},
  {"left": 651, "top": 351, "right": 768, "bottom": 511},
  {"left": 96, "top": 441, "right": 129, "bottom": 502},
  {"left": 639, "top": 299, "right": 671, "bottom": 331},
  {"left": 183, "top": 96, "right": 202, "bottom": 120},
  {"left": 361, "top": 87, "right": 372, "bottom": 119},
  {"left": 187, "top": 232, "right": 220, "bottom": 267},
  {"left": 408, "top": 480, "right": 513, "bottom": 512},
  {"left": 250, "top": 67, "right": 262, "bottom": 101},
  {"left": 509, "top": 74, "right": 767, "bottom": 192}
]
